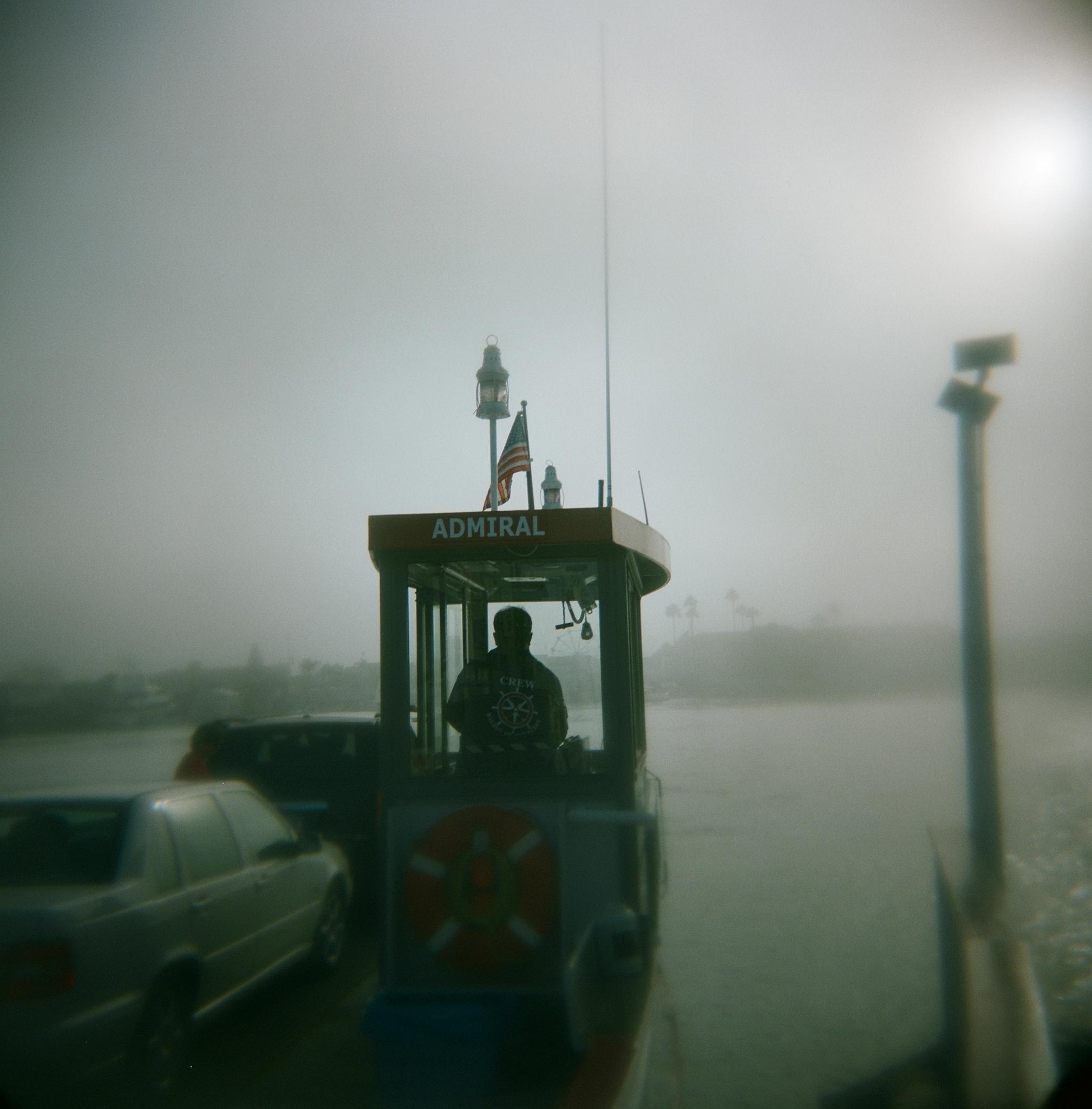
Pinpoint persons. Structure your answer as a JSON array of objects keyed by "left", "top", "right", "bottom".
[{"left": 443, "top": 606, "right": 569, "bottom": 750}]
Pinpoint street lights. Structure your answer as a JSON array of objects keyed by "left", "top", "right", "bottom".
[{"left": 932, "top": 330, "right": 1020, "bottom": 930}]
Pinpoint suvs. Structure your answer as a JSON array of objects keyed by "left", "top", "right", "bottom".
[{"left": 178, "top": 711, "right": 421, "bottom": 874}]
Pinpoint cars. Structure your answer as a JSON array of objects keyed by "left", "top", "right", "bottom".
[{"left": 0, "top": 776, "right": 356, "bottom": 1109}]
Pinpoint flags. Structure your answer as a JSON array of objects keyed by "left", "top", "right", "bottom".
[{"left": 481, "top": 411, "right": 531, "bottom": 512}]
